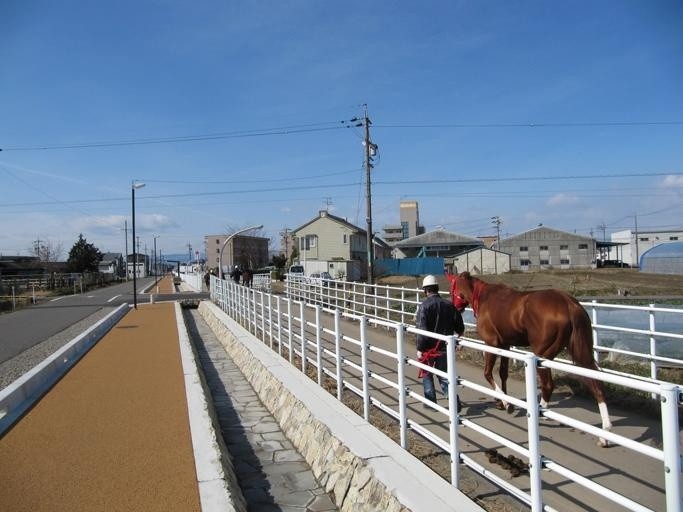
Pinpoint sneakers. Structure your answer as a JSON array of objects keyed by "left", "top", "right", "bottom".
[
  {"left": 423, "top": 404, "right": 438, "bottom": 412},
  {"left": 457, "top": 394, "right": 461, "bottom": 413}
]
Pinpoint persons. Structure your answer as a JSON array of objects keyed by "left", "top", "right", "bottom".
[
  {"left": 415, "top": 275, "right": 465, "bottom": 414},
  {"left": 203, "top": 265, "right": 252, "bottom": 292},
  {"left": 37, "top": 284, "right": 41, "bottom": 289}
]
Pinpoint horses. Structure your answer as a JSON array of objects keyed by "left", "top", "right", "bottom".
[
  {"left": 443, "top": 269, "right": 614, "bottom": 449},
  {"left": 241, "top": 269, "right": 253, "bottom": 293}
]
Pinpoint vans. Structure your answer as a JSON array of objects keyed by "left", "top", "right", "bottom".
[{"left": 288, "top": 266, "right": 305, "bottom": 282}]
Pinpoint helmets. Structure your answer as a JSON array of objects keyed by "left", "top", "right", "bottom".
[{"left": 422, "top": 275, "right": 439, "bottom": 288}]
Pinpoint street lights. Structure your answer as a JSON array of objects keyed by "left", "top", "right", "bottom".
[
  {"left": 132, "top": 181, "right": 148, "bottom": 309},
  {"left": 220, "top": 225, "right": 264, "bottom": 279}
]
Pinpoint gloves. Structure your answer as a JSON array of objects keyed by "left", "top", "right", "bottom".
[{"left": 417, "top": 351, "right": 422, "bottom": 358}]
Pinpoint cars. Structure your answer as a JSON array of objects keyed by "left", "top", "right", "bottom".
[{"left": 309, "top": 272, "right": 331, "bottom": 285}]
